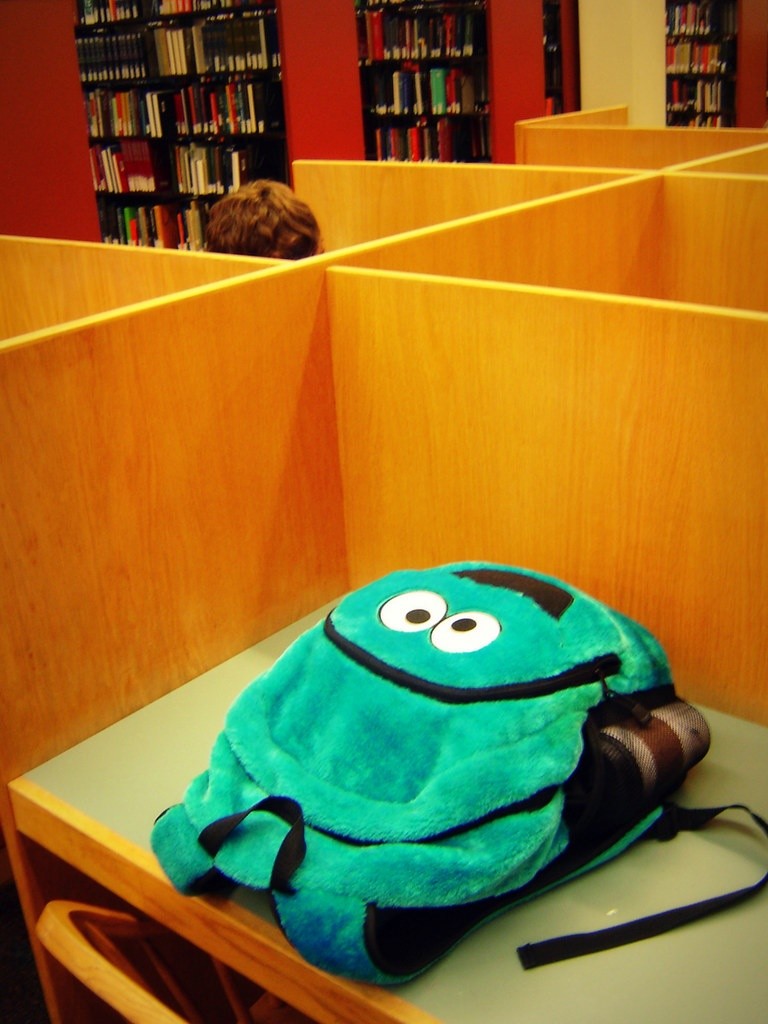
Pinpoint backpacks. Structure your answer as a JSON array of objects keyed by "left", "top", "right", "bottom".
[{"left": 148, "top": 564, "right": 768, "bottom": 990}]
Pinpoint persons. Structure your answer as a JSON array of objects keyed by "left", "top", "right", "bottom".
[{"left": 199, "top": 178, "right": 320, "bottom": 263}]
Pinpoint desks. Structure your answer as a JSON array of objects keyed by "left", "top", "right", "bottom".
[{"left": 8, "top": 591, "right": 768, "bottom": 1024}]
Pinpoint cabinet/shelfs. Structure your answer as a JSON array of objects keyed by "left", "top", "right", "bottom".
[{"left": 0, "top": 0, "right": 768, "bottom": 253}]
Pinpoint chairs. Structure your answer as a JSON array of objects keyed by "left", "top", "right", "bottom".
[{"left": 33, "top": 897, "right": 318, "bottom": 1024}]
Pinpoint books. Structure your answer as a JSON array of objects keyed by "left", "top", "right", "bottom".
[{"left": 75, "top": 1, "right": 732, "bottom": 253}]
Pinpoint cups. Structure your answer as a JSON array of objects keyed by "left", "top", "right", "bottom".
[{"left": 579, "top": 702, "right": 712, "bottom": 842}]
